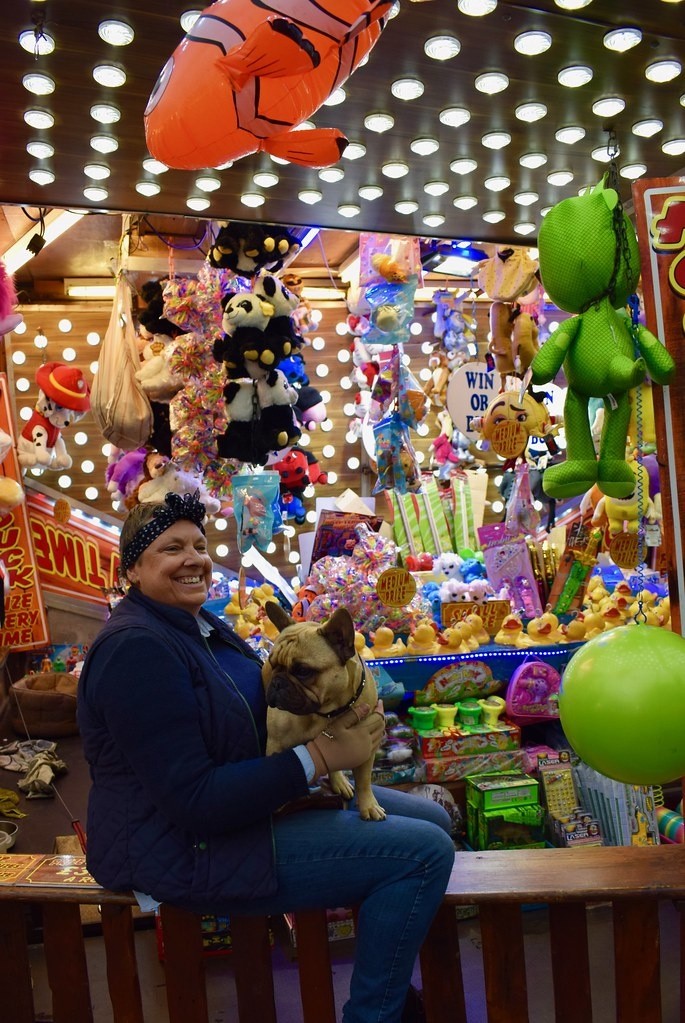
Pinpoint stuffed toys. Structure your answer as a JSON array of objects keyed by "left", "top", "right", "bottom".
[
  {"left": 0, "top": 428, "right": 25, "bottom": 510},
  {"left": 420, "top": 552, "right": 495, "bottom": 627},
  {"left": 15, "top": 360, "right": 93, "bottom": 473},
  {"left": 347, "top": 232, "right": 478, "bottom": 495},
  {"left": 478, "top": 170, "right": 674, "bottom": 539},
  {"left": 104, "top": 221, "right": 326, "bottom": 526},
  {"left": 0, "top": 259, "right": 24, "bottom": 339}
]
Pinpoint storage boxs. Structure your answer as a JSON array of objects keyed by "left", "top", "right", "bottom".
[{"left": 328, "top": 720, "right": 551, "bottom": 853}]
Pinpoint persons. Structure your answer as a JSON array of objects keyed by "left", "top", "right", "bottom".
[{"left": 77, "top": 501, "right": 455, "bottom": 1023}]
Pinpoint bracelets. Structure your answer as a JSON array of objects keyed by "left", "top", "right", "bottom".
[{"left": 313, "top": 740, "right": 330, "bottom": 773}]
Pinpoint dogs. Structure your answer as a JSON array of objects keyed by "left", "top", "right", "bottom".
[{"left": 262, "top": 602, "right": 387, "bottom": 822}]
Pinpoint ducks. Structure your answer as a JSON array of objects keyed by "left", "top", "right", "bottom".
[{"left": 224, "top": 576, "right": 670, "bottom": 661}]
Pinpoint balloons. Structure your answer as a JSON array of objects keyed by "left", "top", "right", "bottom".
[
  {"left": 144, "top": 0, "right": 398, "bottom": 170},
  {"left": 558, "top": 622, "right": 685, "bottom": 786}
]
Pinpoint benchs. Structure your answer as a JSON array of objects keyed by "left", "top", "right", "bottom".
[{"left": 0, "top": 836, "right": 685, "bottom": 1023}]
